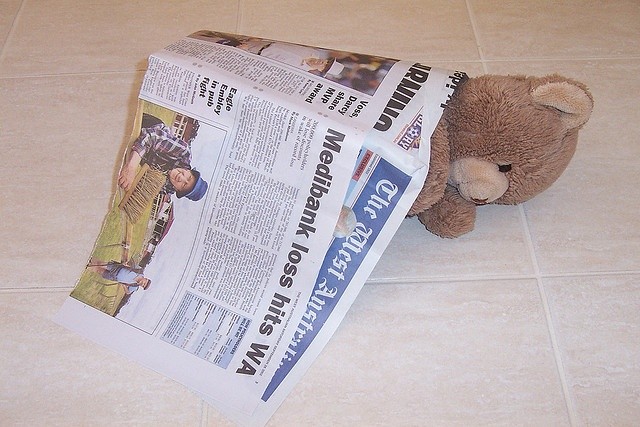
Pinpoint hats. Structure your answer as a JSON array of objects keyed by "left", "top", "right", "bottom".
[
  {"left": 321, "top": 56, "right": 345, "bottom": 78},
  {"left": 176, "top": 170, "right": 208, "bottom": 201},
  {"left": 144, "top": 280, "right": 151, "bottom": 290}
]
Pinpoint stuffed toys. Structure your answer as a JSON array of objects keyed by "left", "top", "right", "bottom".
[{"left": 404, "top": 73, "right": 594, "bottom": 238}]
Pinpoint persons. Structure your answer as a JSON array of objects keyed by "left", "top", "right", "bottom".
[
  {"left": 85, "top": 256, "right": 152, "bottom": 295},
  {"left": 118, "top": 111, "right": 209, "bottom": 203},
  {"left": 333, "top": 205, "right": 357, "bottom": 238},
  {"left": 191, "top": 32, "right": 344, "bottom": 78}
]
